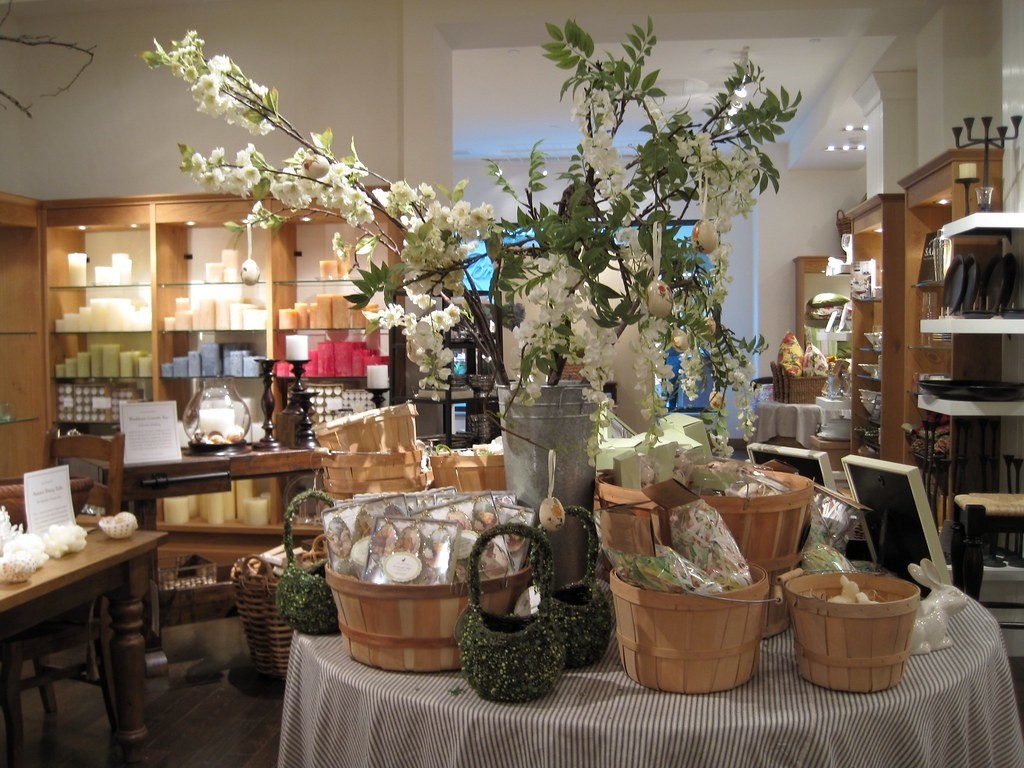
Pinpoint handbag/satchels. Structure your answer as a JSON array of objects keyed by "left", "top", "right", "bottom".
[
  {"left": 530, "top": 505, "right": 612, "bottom": 670},
  {"left": 275, "top": 489, "right": 343, "bottom": 635},
  {"left": 456, "top": 522, "right": 566, "bottom": 703}
]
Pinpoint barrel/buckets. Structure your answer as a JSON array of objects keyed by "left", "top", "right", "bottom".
[
  {"left": 307, "top": 405, "right": 533, "bottom": 674},
  {"left": 593, "top": 462, "right": 922, "bottom": 695}
]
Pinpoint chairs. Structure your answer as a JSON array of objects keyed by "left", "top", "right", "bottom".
[{"left": 1, "top": 431, "right": 125, "bottom": 760}]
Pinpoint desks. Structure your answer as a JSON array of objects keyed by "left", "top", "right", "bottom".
[
  {"left": 0, "top": 519, "right": 168, "bottom": 762},
  {"left": 277, "top": 557, "right": 1024, "bottom": 768},
  {"left": 755, "top": 398, "right": 820, "bottom": 450}
]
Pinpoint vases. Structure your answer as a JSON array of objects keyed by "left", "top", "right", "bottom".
[{"left": 490, "top": 381, "right": 602, "bottom": 507}]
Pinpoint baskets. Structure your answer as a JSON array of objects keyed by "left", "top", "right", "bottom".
[
  {"left": 228, "top": 534, "right": 328, "bottom": 683},
  {"left": 769, "top": 361, "right": 826, "bottom": 405}
]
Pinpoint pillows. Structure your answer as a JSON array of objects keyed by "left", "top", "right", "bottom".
[
  {"left": 809, "top": 306, "right": 852, "bottom": 321},
  {"left": 777, "top": 330, "right": 803, "bottom": 377},
  {"left": 802, "top": 341, "right": 827, "bottom": 376},
  {"left": 807, "top": 293, "right": 850, "bottom": 308},
  {"left": 901, "top": 422, "right": 950, "bottom": 459}
]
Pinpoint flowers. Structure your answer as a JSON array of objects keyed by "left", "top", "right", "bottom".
[{"left": 132, "top": 14, "right": 802, "bottom": 496}]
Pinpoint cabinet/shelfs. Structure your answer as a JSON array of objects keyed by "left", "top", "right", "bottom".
[
  {"left": 897, "top": 146, "right": 1024, "bottom": 532},
  {"left": 845, "top": 194, "right": 904, "bottom": 465},
  {"left": 39, "top": 222, "right": 396, "bottom": 456},
  {"left": 0, "top": 223, "right": 48, "bottom": 481}
]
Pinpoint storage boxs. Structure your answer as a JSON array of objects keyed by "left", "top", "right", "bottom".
[{"left": 158, "top": 551, "right": 235, "bottom": 625}]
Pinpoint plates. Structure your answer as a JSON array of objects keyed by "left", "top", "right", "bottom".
[
  {"left": 941, "top": 253, "right": 1017, "bottom": 316},
  {"left": 917, "top": 379, "right": 1024, "bottom": 402}
]
